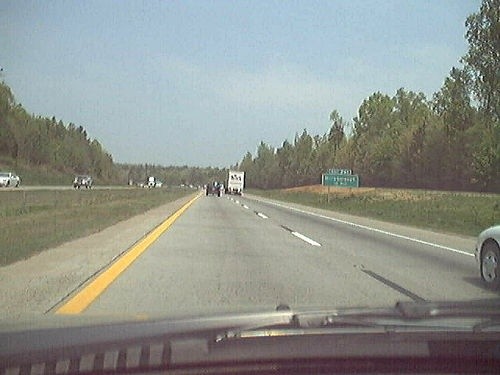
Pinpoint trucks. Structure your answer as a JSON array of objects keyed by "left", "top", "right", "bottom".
[{"left": 224, "top": 170, "right": 245, "bottom": 195}]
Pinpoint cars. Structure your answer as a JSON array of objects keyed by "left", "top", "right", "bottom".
[
  {"left": 474, "top": 225, "right": 499, "bottom": 291},
  {"left": 0, "top": 172, "right": 22, "bottom": 188}
]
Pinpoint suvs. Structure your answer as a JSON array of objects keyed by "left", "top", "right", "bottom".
[
  {"left": 206, "top": 180, "right": 221, "bottom": 197},
  {"left": 72, "top": 174, "right": 94, "bottom": 190}
]
